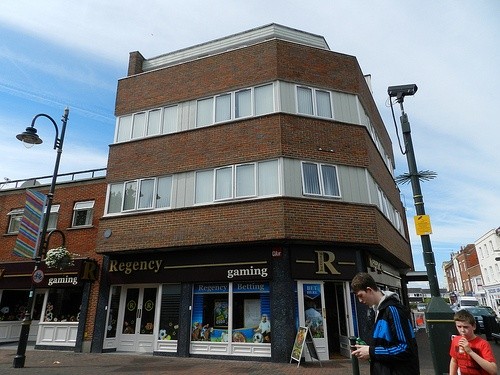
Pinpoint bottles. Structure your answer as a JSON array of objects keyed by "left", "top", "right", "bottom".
[
  {"left": 459, "top": 335, "right": 466, "bottom": 354},
  {"left": 356, "top": 338, "right": 373, "bottom": 363}
]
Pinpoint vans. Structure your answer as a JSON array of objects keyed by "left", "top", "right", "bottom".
[{"left": 457, "top": 296, "right": 479, "bottom": 310}]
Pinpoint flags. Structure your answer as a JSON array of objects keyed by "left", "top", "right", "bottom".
[{"left": 13, "top": 187, "right": 46, "bottom": 260}]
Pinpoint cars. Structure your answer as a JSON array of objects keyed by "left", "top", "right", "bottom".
[
  {"left": 465, "top": 307, "right": 500, "bottom": 333},
  {"left": 417, "top": 303, "right": 428, "bottom": 312}
]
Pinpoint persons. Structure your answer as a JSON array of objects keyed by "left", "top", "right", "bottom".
[
  {"left": 350, "top": 272, "right": 420, "bottom": 375},
  {"left": 305, "top": 302, "right": 322, "bottom": 328},
  {"left": 449, "top": 309, "right": 498, "bottom": 375},
  {"left": 253, "top": 313, "right": 271, "bottom": 343}
]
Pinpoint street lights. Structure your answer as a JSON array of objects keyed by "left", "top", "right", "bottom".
[
  {"left": 12, "top": 105, "right": 70, "bottom": 367},
  {"left": 388, "top": 82, "right": 459, "bottom": 375}
]
